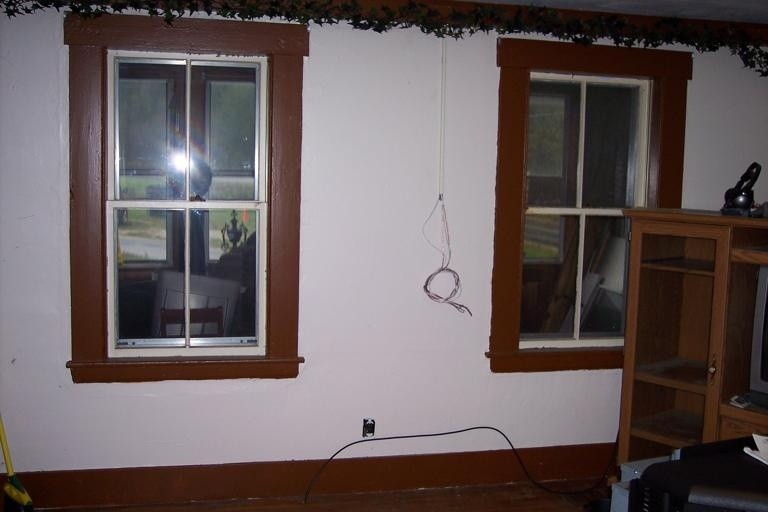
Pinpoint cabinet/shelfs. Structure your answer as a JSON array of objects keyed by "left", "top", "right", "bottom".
[{"left": 605, "top": 206, "right": 768, "bottom": 489}]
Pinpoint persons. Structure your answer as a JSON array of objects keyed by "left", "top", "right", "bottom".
[{"left": 165, "top": 129, "right": 215, "bottom": 278}]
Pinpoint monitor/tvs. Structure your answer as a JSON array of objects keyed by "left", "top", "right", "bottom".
[{"left": 745, "top": 266, "right": 768, "bottom": 409}]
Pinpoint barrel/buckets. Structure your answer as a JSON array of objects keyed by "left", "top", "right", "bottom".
[{"left": 152, "top": 272, "right": 240, "bottom": 339}]
